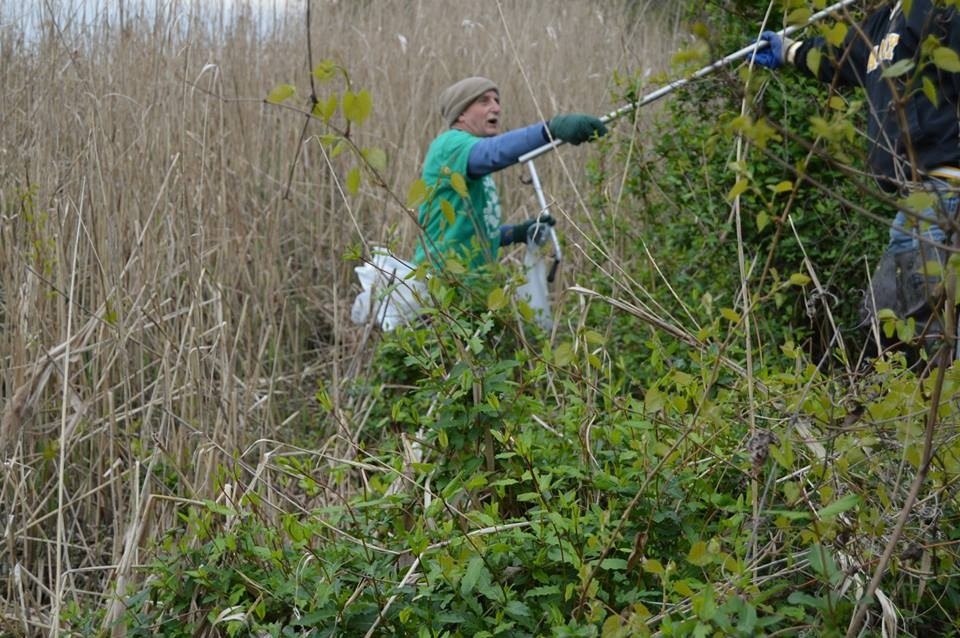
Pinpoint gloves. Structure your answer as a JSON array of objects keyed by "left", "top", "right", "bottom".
[
  {"left": 746, "top": 30, "right": 795, "bottom": 69},
  {"left": 512, "top": 213, "right": 556, "bottom": 248},
  {"left": 548, "top": 115, "right": 609, "bottom": 146}
]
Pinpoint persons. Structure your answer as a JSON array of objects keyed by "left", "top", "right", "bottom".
[
  {"left": 747, "top": 0, "right": 960, "bottom": 425},
  {"left": 409, "top": 77, "right": 608, "bottom": 320}
]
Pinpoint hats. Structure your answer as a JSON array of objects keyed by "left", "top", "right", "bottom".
[{"left": 437, "top": 77, "right": 501, "bottom": 129}]
